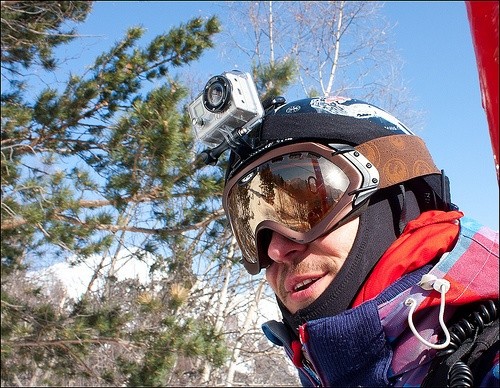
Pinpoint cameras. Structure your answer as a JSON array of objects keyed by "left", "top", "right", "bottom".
[{"left": 186, "top": 70, "right": 266, "bottom": 150}]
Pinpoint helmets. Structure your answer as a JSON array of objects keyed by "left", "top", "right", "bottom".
[{"left": 225, "top": 95, "right": 441, "bottom": 212}]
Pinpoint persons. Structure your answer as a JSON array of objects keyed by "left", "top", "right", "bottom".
[
  {"left": 307, "top": 205, "right": 322, "bottom": 228},
  {"left": 222, "top": 96, "right": 500, "bottom": 387}
]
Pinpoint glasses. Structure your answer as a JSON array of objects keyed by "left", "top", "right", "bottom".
[{"left": 221, "top": 134, "right": 443, "bottom": 275}]
